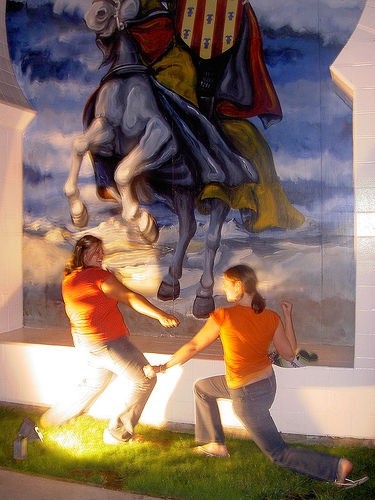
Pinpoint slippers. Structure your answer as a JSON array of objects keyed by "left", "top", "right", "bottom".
[
  {"left": 334, "top": 476, "right": 368, "bottom": 490},
  {"left": 198, "top": 445, "right": 230, "bottom": 458}
]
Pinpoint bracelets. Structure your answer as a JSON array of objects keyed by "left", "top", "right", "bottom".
[{"left": 159, "top": 364, "right": 166, "bottom": 374}]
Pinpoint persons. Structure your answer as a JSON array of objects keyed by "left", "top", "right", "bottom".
[
  {"left": 10, "top": 235, "right": 180, "bottom": 459},
  {"left": 143, "top": 262, "right": 353, "bottom": 490}
]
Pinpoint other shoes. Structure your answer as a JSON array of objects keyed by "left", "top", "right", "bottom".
[
  {"left": 102, "top": 429, "right": 144, "bottom": 445},
  {"left": 39, "top": 411, "right": 67, "bottom": 429}
]
